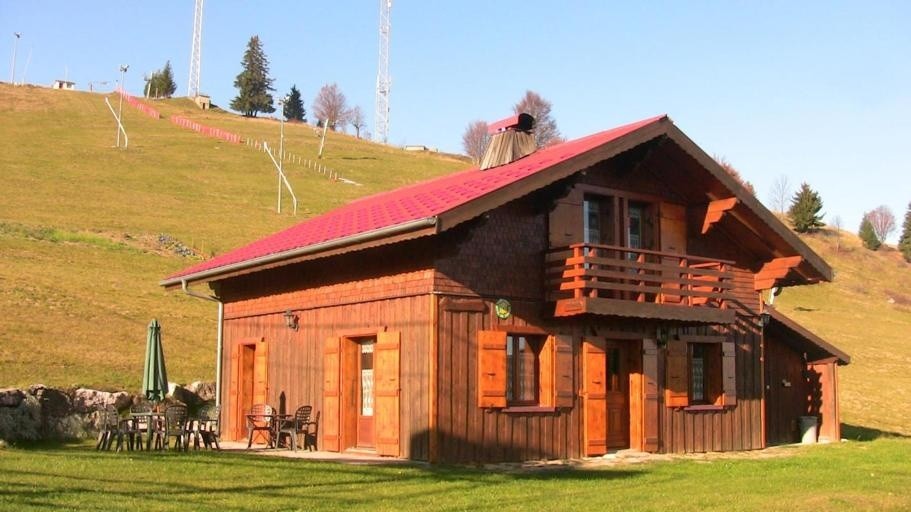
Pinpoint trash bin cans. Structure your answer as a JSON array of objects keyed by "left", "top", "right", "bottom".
[{"left": 799, "top": 417, "right": 817, "bottom": 444}]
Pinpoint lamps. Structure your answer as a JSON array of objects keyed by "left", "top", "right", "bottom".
[
  {"left": 657, "top": 322, "right": 671, "bottom": 345},
  {"left": 283, "top": 308, "right": 298, "bottom": 329}
]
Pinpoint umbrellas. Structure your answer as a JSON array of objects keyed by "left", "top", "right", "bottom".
[{"left": 142, "top": 319, "right": 170, "bottom": 413}]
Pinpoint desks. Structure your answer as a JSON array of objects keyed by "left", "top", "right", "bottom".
[{"left": 263, "top": 414, "right": 292, "bottom": 448}]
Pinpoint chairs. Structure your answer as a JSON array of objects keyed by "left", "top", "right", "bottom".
[
  {"left": 95, "top": 398, "right": 221, "bottom": 452},
  {"left": 275, "top": 406, "right": 312, "bottom": 453},
  {"left": 246, "top": 404, "right": 273, "bottom": 449}
]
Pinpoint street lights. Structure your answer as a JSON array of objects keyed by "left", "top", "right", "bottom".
[
  {"left": 11, "top": 30, "right": 24, "bottom": 83},
  {"left": 115, "top": 62, "right": 132, "bottom": 149},
  {"left": 272, "top": 94, "right": 294, "bottom": 216}
]
